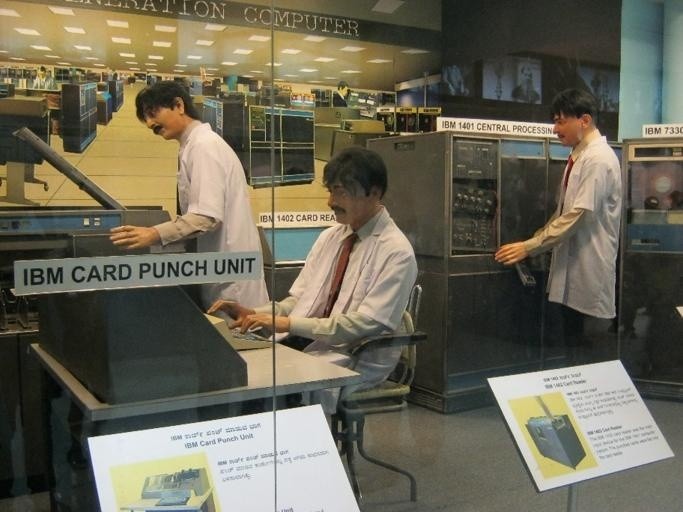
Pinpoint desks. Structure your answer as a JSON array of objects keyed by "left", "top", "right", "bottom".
[{"left": 30, "top": 322, "right": 361, "bottom": 512}]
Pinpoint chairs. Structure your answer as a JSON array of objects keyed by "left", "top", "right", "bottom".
[{"left": 331, "top": 285, "right": 428, "bottom": 507}]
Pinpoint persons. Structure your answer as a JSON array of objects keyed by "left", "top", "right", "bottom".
[
  {"left": 495, "top": 88, "right": 623, "bottom": 362},
  {"left": 33, "top": 66, "right": 58, "bottom": 90},
  {"left": 332, "top": 80, "right": 349, "bottom": 107},
  {"left": 206, "top": 147, "right": 417, "bottom": 430},
  {"left": 108, "top": 82, "right": 270, "bottom": 332}
]
